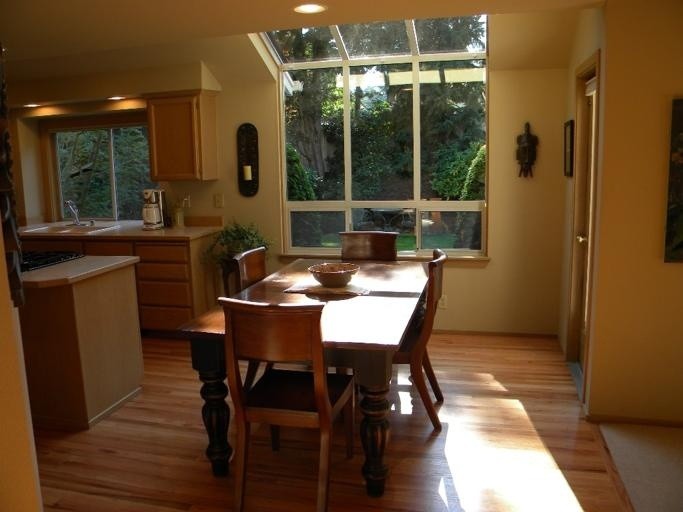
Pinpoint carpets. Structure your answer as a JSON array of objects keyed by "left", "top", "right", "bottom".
[{"left": 598, "top": 421, "right": 682, "bottom": 512}]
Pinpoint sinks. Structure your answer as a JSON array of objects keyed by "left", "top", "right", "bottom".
[{"left": 22, "top": 224, "right": 121, "bottom": 234}]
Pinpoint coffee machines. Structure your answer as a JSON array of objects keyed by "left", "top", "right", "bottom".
[{"left": 141, "top": 189, "right": 166, "bottom": 230}]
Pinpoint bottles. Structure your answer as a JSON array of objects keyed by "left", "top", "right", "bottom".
[{"left": 173, "top": 207, "right": 185, "bottom": 228}]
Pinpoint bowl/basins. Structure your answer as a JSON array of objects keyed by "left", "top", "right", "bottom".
[{"left": 306, "top": 263, "right": 359, "bottom": 287}]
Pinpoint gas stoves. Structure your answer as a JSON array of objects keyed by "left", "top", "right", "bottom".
[{"left": 19, "top": 250, "right": 84, "bottom": 271}]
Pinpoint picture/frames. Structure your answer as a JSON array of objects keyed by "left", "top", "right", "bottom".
[{"left": 563, "top": 118, "right": 574, "bottom": 177}]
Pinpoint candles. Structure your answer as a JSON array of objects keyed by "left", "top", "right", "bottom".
[{"left": 242, "top": 165, "right": 252, "bottom": 182}]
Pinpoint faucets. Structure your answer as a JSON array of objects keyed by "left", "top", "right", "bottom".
[{"left": 64, "top": 200, "right": 81, "bottom": 226}]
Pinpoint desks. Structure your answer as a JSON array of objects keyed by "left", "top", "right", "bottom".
[{"left": 20, "top": 253, "right": 144, "bottom": 431}]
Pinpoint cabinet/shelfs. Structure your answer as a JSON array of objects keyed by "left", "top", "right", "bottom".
[
  {"left": 135, "top": 230, "right": 228, "bottom": 334},
  {"left": 82, "top": 241, "right": 136, "bottom": 256},
  {"left": 147, "top": 89, "right": 219, "bottom": 182}
]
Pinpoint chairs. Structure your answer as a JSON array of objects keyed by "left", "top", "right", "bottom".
[
  {"left": 232, "top": 244, "right": 274, "bottom": 392},
  {"left": 391, "top": 248, "right": 447, "bottom": 432},
  {"left": 337, "top": 230, "right": 400, "bottom": 261},
  {"left": 216, "top": 295, "right": 356, "bottom": 511}
]
